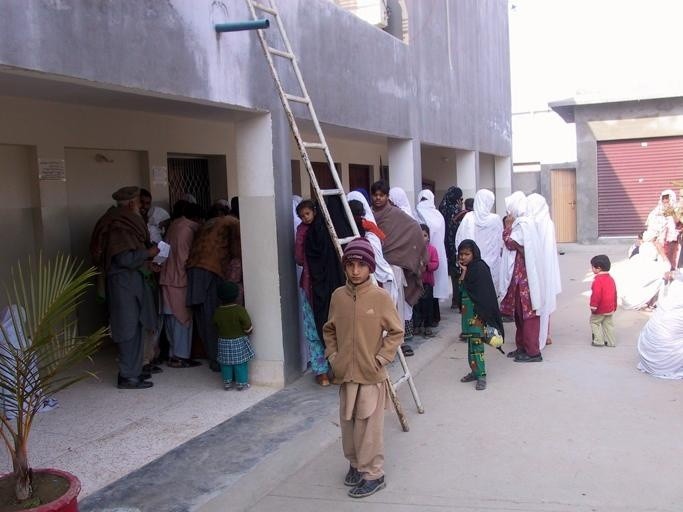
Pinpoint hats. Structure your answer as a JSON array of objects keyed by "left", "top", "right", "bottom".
[
  {"left": 342, "top": 237, "right": 375, "bottom": 274},
  {"left": 111, "top": 186, "right": 140, "bottom": 200}
]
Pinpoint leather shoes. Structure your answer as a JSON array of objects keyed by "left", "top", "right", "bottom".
[
  {"left": 170, "top": 355, "right": 202, "bottom": 368},
  {"left": 118, "top": 355, "right": 162, "bottom": 389}
]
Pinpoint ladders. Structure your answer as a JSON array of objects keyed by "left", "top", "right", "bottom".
[{"left": 245, "top": 0, "right": 425, "bottom": 433}]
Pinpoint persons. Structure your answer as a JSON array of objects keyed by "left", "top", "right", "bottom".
[
  {"left": 292, "top": 181, "right": 563, "bottom": 390},
  {"left": 613, "top": 190, "right": 682, "bottom": 379},
  {"left": 89, "top": 186, "right": 253, "bottom": 390},
  {"left": 323, "top": 238, "right": 403, "bottom": 497},
  {"left": 590, "top": 254, "right": 618, "bottom": 346}
]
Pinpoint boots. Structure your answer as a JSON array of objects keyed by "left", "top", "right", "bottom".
[{"left": 203, "top": 340, "right": 220, "bottom": 372}]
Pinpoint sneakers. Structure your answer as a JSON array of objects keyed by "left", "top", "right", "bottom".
[
  {"left": 460, "top": 373, "right": 477, "bottom": 382},
  {"left": 513, "top": 352, "right": 542, "bottom": 362},
  {"left": 507, "top": 348, "right": 525, "bottom": 358},
  {"left": 344, "top": 464, "right": 364, "bottom": 485},
  {"left": 476, "top": 380, "right": 486, "bottom": 390},
  {"left": 236, "top": 383, "right": 250, "bottom": 391},
  {"left": 348, "top": 475, "right": 386, "bottom": 498},
  {"left": 316, "top": 373, "right": 330, "bottom": 386}
]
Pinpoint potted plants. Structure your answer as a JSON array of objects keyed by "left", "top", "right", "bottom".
[{"left": 0, "top": 248, "right": 111, "bottom": 512}]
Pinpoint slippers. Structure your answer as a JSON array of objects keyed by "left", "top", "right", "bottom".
[{"left": 401, "top": 345, "right": 413, "bottom": 356}]
